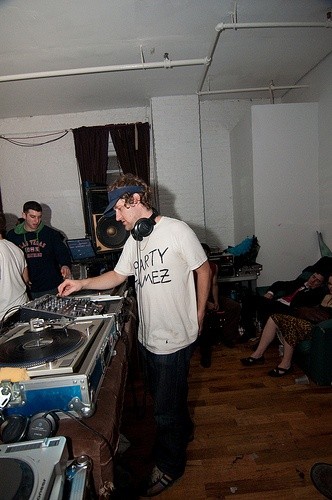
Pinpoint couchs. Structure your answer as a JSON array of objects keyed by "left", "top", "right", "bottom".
[{"left": 245, "top": 256, "right": 332, "bottom": 386}]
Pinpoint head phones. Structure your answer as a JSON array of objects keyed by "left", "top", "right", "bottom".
[
  {"left": 130, "top": 207, "right": 159, "bottom": 241},
  {"left": 0, "top": 411, "right": 60, "bottom": 444}
]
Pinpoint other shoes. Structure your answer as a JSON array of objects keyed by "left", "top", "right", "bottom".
[
  {"left": 310, "top": 462, "right": 332, "bottom": 500},
  {"left": 240, "top": 355, "right": 265, "bottom": 366},
  {"left": 200, "top": 350, "right": 212, "bottom": 368},
  {"left": 220, "top": 296, "right": 240, "bottom": 313},
  {"left": 138, "top": 464, "right": 184, "bottom": 497},
  {"left": 223, "top": 339, "right": 235, "bottom": 348},
  {"left": 267, "top": 366, "right": 293, "bottom": 377}
]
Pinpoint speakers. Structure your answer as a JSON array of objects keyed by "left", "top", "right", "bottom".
[{"left": 91, "top": 213, "right": 130, "bottom": 255}]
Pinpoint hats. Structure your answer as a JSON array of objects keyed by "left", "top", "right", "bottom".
[{"left": 102, "top": 186, "right": 147, "bottom": 217}]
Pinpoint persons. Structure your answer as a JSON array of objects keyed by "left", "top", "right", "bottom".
[
  {"left": 195, "top": 243, "right": 220, "bottom": 369},
  {"left": 240, "top": 274, "right": 332, "bottom": 377},
  {"left": 0, "top": 212, "right": 31, "bottom": 323},
  {"left": 57, "top": 174, "right": 212, "bottom": 496},
  {"left": 7, "top": 200, "right": 72, "bottom": 301},
  {"left": 241, "top": 271, "right": 327, "bottom": 352}
]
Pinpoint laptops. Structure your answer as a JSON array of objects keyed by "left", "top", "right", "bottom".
[{"left": 66, "top": 238, "right": 104, "bottom": 263}]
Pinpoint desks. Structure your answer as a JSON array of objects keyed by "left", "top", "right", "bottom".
[
  {"left": 57, "top": 295, "right": 138, "bottom": 500},
  {"left": 218, "top": 274, "right": 258, "bottom": 292}
]
